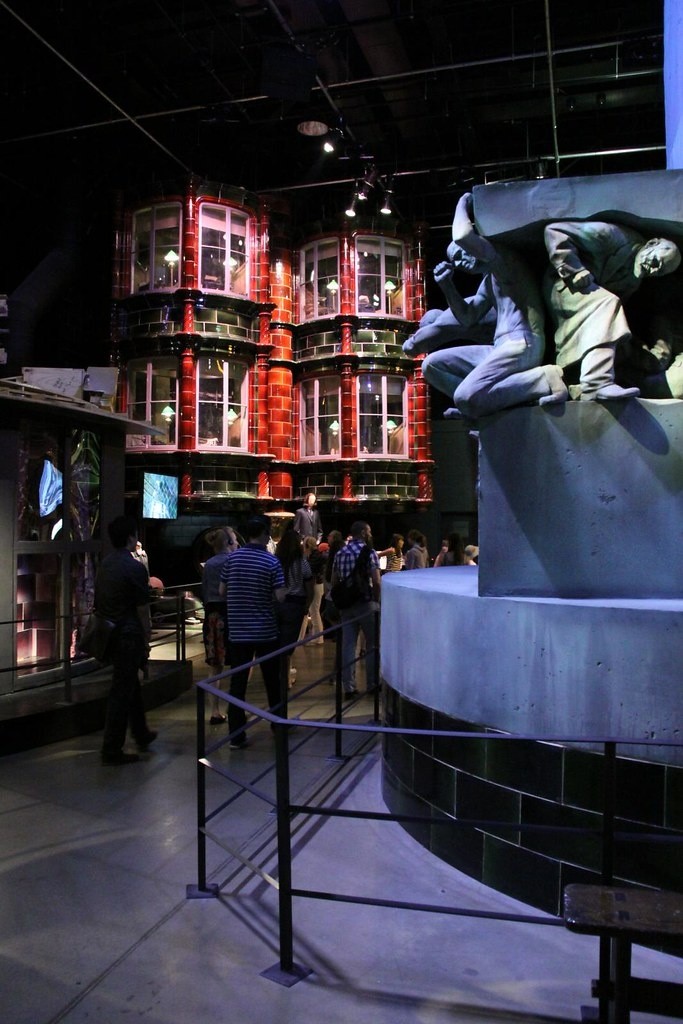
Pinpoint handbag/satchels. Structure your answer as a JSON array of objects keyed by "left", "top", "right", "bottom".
[
  {"left": 77, "top": 611, "right": 117, "bottom": 664},
  {"left": 330, "top": 545, "right": 373, "bottom": 610}
]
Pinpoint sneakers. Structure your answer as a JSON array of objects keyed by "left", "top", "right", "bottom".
[
  {"left": 345, "top": 690, "right": 361, "bottom": 700},
  {"left": 329, "top": 678, "right": 336, "bottom": 686},
  {"left": 303, "top": 640, "right": 326, "bottom": 648},
  {"left": 366, "top": 689, "right": 385, "bottom": 702}
]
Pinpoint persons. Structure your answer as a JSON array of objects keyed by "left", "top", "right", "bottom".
[
  {"left": 292, "top": 493, "right": 324, "bottom": 546},
  {"left": 541, "top": 213, "right": 680, "bottom": 409},
  {"left": 130, "top": 540, "right": 159, "bottom": 636},
  {"left": 198, "top": 486, "right": 480, "bottom": 751},
  {"left": 401, "top": 187, "right": 572, "bottom": 425},
  {"left": 89, "top": 515, "right": 166, "bottom": 768}
]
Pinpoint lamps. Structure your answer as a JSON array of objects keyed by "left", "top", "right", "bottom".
[
  {"left": 379, "top": 193, "right": 393, "bottom": 215},
  {"left": 228, "top": 409, "right": 239, "bottom": 425},
  {"left": 329, "top": 419, "right": 339, "bottom": 436},
  {"left": 323, "top": 130, "right": 341, "bottom": 152},
  {"left": 358, "top": 184, "right": 371, "bottom": 201},
  {"left": 161, "top": 405, "right": 175, "bottom": 422},
  {"left": 326, "top": 280, "right": 339, "bottom": 310},
  {"left": 164, "top": 250, "right": 178, "bottom": 286},
  {"left": 385, "top": 173, "right": 396, "bottom": 194},
  {"left": 384, "top": 281, "right": 397, "bottom": 314},
  {"left": 385, "top": 420, "right": 397, "bottom": 434},
  {"left": 334, "top": 114, "right": 347, "bottom": 134},
  {"left": 341, "top": 179, "right": 360, "bottom": 216},
  {"left": 222, "top": 256, "right": 238, "bottom": 266},
  {"left": 364, "top": 166, "right": 378, "bottom": 187}
]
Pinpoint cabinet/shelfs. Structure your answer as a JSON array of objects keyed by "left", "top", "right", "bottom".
[
  {"left": 228, "top": 412, "right": 241, "bottom": 447},
  {"left": 305, "top": 426, "right": 322, "bottom": 456},
  {"left": 231, "top": 262, "right": 246, "bottom": 295},
  {"left": 389, "top": 423, "right": 404, "bottom": 454},
  {"left": 392, "top": 285, "right": 403, "bottom": 315}
]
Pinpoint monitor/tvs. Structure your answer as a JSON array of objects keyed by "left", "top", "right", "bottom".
[{"left": 143, "top": 472, "right": 179, "bottom": 519}]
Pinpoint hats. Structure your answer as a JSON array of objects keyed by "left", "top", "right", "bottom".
[
  {"left": 449, "top": 532, "right": 464, "bottom": 546},
  {"left": 392, "top": 534, "right": 404, "bottom": 545},
  {"left": 464, "top": 544, "right": 479, "bottom": 560}
]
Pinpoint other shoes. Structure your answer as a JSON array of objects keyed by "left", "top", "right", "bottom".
[
  {"left": 229, "top": 743, "right": 248, "bottom": 751},
  {"left": 135, "top": 731, "right": 158, "bottom": 752},
  {"left": 210, "top": 715, "right": 226, "bottom": 725},
  {"left": 288, "top": 680, "right": 296, "bottom": 690},
  {"left": 102, "top": 750, "right": 140, "bottom": 766},
  {"left": 270, "top": 721, "right": 295, "bottom": 736}
]
